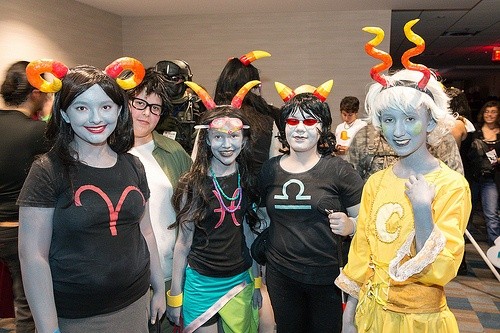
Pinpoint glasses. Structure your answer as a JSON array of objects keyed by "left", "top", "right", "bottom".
[
  {"left": 128, "top": 96, "right": 166, "bottom": 116},
  {"left": 284, "top": 117, "right": 323, "bottom": 126}
]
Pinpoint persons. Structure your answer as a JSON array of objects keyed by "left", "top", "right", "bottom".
[
  {"left": 333, "top": 67, "right": 472, "bottom": 333},
  {"left": 122, "top": 66, "right": 194, "bottom": 332},
  {"left": 470, "top": 100, "right": 500, "bottom": 246},
  {"left": 166, "top": 104, "right": 269, "bottom": 333},
  {"left": 258, "top": 93, "right": 366, "bottom": 333},
  {"left": 144, "top": 56, "right": 465, "bottom": 333},
  {"left": 0, "top": 61, "right": 54, "bottom": 333},
  {"left": 14, "top": 64, "right": 168, "bottom": 333}
]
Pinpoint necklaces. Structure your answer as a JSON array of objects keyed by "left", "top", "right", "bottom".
[
  {"left": 207, "top": 161, "right": 240, "bottom": 200},
  {"left": 212, "top": 178, "right": 242, "bottom": 212}
]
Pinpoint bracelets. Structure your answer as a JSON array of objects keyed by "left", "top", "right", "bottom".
[
  {"left": 167, "top": 290, "right": 182, "bottom": 307},
  {"left": 253, "top": 277, "right": 262, "bottom": 288},
  {"left": 349, "top": 217, "right": 356, "bottom": 236}
]
